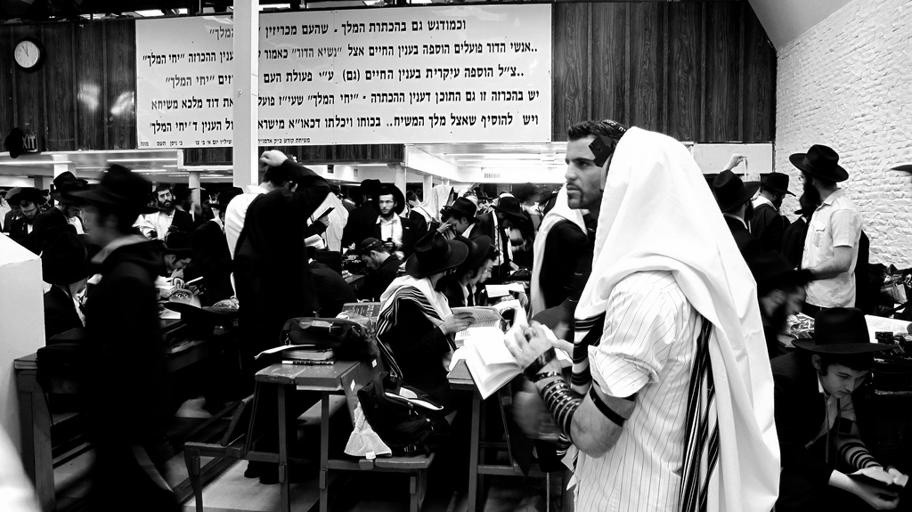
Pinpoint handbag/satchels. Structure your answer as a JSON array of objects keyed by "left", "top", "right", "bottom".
[
  {"left": 358, "top": 372, "right": 452, "bottom": 452},
  {"left": 167, "top": 278, "right": 201, "bottom": 308},
  {"left": 284, "top": 317, "right": 381, "bottom": 362}
]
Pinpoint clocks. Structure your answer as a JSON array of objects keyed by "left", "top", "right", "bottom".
[{"left": 13, "top": 40, "right": 44, "bottom": 71}]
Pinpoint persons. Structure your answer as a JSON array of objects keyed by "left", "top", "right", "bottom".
[
  {"left": 522, "top": 118, "right": 629, "bottom": 344},
  {"left": 1, "top": 143, "right": 587, "bottom": 441},
  {"left": 55, "top": 158, "right": 185, "bottom": 511},
  {"left": 768, "top": 307, "right": 911, "bottom": 512},
  {"left": 503, "top": 125, "right": 783, "bottom": 510},
  {"left": 710, "top": 144, "right": 862, "bottom": 367}
]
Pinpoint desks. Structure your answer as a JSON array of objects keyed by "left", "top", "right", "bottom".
[{"left": 16, "top": 257, "right": 361, "bottom": 511}]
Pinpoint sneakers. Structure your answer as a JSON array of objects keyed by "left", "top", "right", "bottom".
[{"left": 243, "top": 458, "right": 319, "bottom": 485}]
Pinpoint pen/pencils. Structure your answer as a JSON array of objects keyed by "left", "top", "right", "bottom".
[{"left": 282, "top": 361, "right": 334, "bottom": 365}]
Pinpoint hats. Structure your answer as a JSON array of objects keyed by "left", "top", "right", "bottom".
[
  {"left": 358, "top": 237, "right": 382, "bottom": 256},
  {"left": 52, "top": 163, "right": 158, "bottom": 215},
  {"left": 711, "top": 169, "right": 761, "bottom": 213},
  {"left": 165, "top": 301, "right": 238, "bottom": 342},
  {"left": 490, "top": 193, "right": 528, "bottom": 222},
  {"left": 361, "top": 178, "right": 405, "bottom": 215},
  {"left": 758, "top": 172, "right": 797, "bottom": 197},
  {"left": 789, "top": 144, "right": 849, "bottom": 181},
  {"left": 444, "top": 197, "right": 481, "bottom": 224},
  {"left": 211, "top": 187, "right": 243, "bottom": 211},
  {"left": 406, "top": 230, "right": 492, "bottom": 279},
  {"left": 791, "top": 308, "right": 900, "bottom": 355}
]
